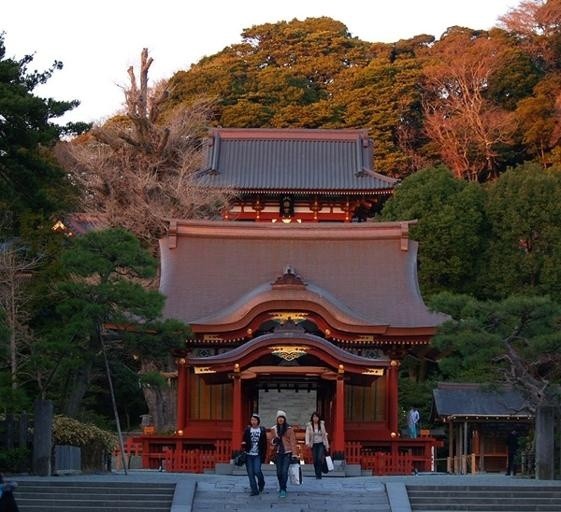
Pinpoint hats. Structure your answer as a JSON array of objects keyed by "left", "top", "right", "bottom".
[{"left": 277, "top": 410, "right": 286, "bottom": 418}]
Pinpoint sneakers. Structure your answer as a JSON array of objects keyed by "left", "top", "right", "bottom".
[{"left": 279, "top": 489, "right": 286, "bottom": 498}]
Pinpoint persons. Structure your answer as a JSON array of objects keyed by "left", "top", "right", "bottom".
[
  {"left": 409, "top": 406, "right": 420, "bottom": 438},
  {"left": 505, "top": 428, "right": 518, "bottom": 476},
  {"left": 241, "top": 414, "right": 268, "bottom": 496},
  {"left": 305, "top": 411, "right": 329, "bottom": 479},
  {"left": 270, "top": 410, "right": 298, "bottom": 498}
]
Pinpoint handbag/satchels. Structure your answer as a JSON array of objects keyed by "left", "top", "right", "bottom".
[
  {"left": 321, "top": 455, "right": 328, "bottom": 473},
  {"left": 270, "top": 446, "right": 278, "bottom": 464},
  {"left": 288, "top": 461, "right": 303, "bottom": 486},
  {"left": 325, "top": 456, "right": 334, "bottom": 471},
  {"left": 233, "top": 448, "right": 248, "bottom": 466}
]
